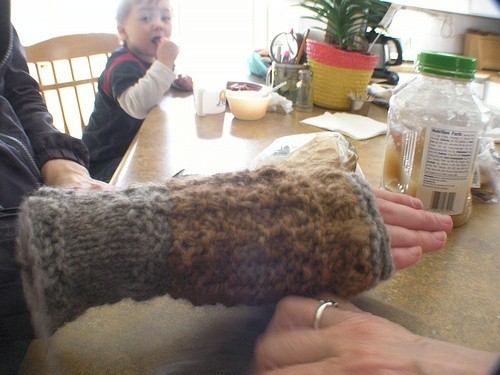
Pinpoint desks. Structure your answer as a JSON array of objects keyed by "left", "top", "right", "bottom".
[{"left": 17, "top": 62, "right": 500, "bottom": 375}]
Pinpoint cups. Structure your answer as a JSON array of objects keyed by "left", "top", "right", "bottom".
[
  {"left": 267, "top": 63, "right": 309, "bottom": 107},
  {"left": 226, "top": 82, "right": 270, "bottom": 120},
  {"left": 194, "top": 84, "right": 226, "bottom": 116},
  {"left": 350, "top": 98, "right": 372, "bottom": 116}
]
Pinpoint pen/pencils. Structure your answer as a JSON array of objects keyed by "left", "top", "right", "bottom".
[
  {"left": 299, "top": 53, "right": 307, "bottom": 65},
  {"left": 283, "top": 50, "right": 290, "bottom": 63},
  {"left": 295, "top": 29, "right": 310, "bottom": 65},
  {"left": 276, "top": 46, "right": 281, "bottom": 62}
]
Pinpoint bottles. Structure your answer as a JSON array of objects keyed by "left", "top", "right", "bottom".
[
  {"left": 382, "top": 51, "right": 479, "bottom": 227},
  {"left": 295, "top": 69, "right": 314, "bottom": 111}
]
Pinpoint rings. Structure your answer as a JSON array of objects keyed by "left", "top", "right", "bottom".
[{"left": 313, "top": 299, "right": 338, "bottom": 328}]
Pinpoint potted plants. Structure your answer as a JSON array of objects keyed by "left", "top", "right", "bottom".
[{"left": 290, "top": 0, "right": 389, "bottom": 110}]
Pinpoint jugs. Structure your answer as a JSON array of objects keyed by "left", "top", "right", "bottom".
[{"left": 367, "top": 27, "right": 402, "bottom": 70}]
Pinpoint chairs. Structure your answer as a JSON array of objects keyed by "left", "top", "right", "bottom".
[{"left": 23, "top": 33, "right": 118, "bottom": 139}]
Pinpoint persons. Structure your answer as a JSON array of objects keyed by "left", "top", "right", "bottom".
[
  {"left": 0, "top": 0, "right": 500, "bottom": 375},
  {"left": 83, "top": 0, "right": 193, "bottom": 182}
]
